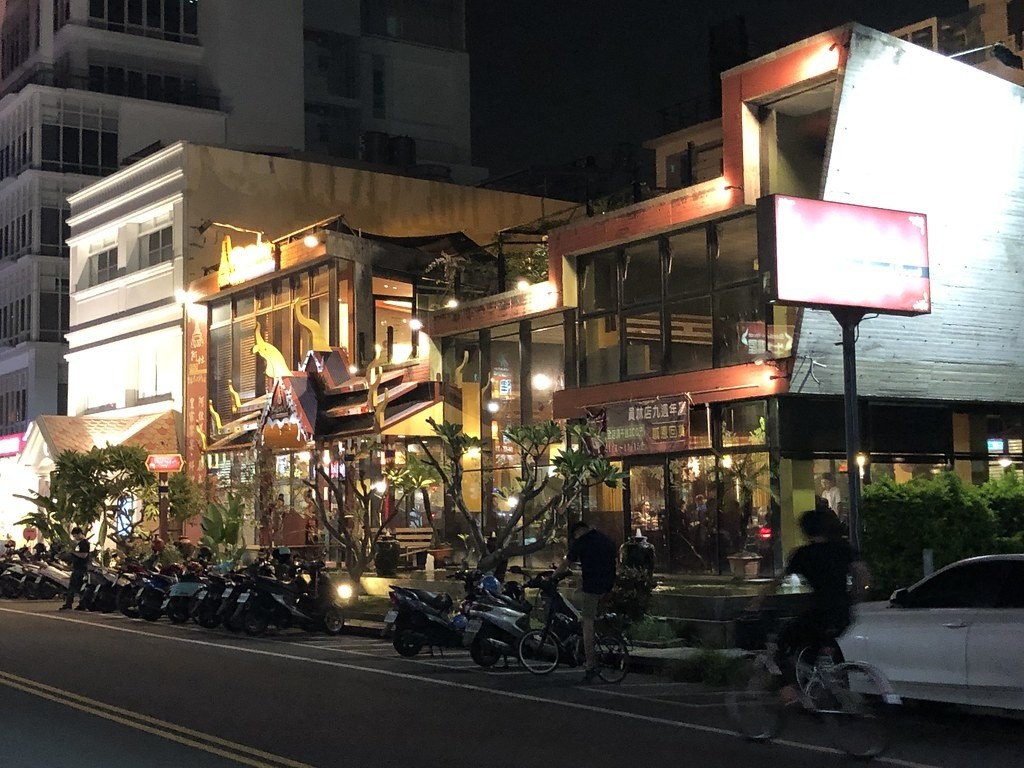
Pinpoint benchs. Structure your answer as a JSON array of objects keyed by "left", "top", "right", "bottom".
[{"left": 363, "top": 526, "right": 434, "bottom": 567}]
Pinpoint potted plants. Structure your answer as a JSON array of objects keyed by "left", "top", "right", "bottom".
[
  {"left": 389, "top": 470, "right": 454, "bottom": 563},
  {"left": 704, "top": 447, "right": 783, "bottom": 581},
  {"left": 166, "top": 472, "right": 206, "bottom": 557}
]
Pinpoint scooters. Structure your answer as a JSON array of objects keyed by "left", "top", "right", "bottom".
[
  {"left": 380, "top": 568, "right": 502, "bottom": 659},
  {"left": 0, "top": 535, "right": 106, "bottom": 600},
  {"left": 462, "top": 566, "right": 582, "bottom": 669},
  {"left": 79, "top": 537, "right": 345, "bottom": 638}
]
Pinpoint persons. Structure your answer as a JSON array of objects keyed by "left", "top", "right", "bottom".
[
  {"left": 632, "top": 500, "right": 652, "bottom": 525},
  {"left": 547, "top": 521, "right": 616, "bottom": 686},
  {"left": 740, "top": 510, "right": 853, "bottom": 731},
  {"left": 60, "top": 527, "right": 90, "bottom": 610},
  {"left": 677, "top": 494, "right": 744, "bottom": 568},
  {"left": 821, "top": 474, "right": 841, "bottom": 519}
]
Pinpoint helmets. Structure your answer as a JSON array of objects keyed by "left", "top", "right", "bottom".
[
  {"left": 503, "top": 581, "right": 524, "bottom": 600},
  {"left": 178, "top": 539, "right": 195, "bottom": 557},
  {"left": 151, "top": 538, "right": 164, "bottom": 552},
  {"left": 200, "top": 545, "right": 214, "bottom": 557},
  {"left": 273, "top": 546, "right": 291, "bottom": 564},
  {"left": 480, "top": 576, "right": 500, "bottom": 594},
  {"left": 34, "top": 543, "right": 45, "bottom": 551}
]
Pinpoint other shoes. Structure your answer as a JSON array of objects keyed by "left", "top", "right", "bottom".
[
  {"left": 59, "top": 604, "right": 72, "bottom": 611},
  {"left": 771, "top": 690, "right": 796, "bottom": 709},
  {"left": 74, "top": 606, "right": 85, "bottom": 611},
  {"left": 583, "top": 665, "right": 601, "bottom": 679}
]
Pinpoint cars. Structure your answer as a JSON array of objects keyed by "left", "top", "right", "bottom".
[{"left": 787, "top": 554, "right": 1024, "bottom": 723}]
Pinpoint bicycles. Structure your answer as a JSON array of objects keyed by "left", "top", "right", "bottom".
[
  {"left": 721, "top": 605, "right": 899, "bottom": 760},
  {"left": 519, "top": 575, "right": 632, "bottom": 684}
]
